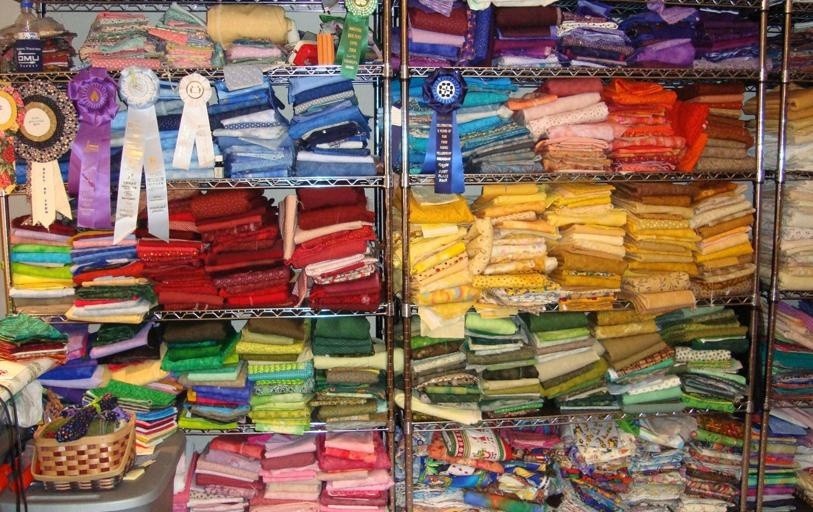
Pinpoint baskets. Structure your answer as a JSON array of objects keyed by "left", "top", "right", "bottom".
[{"left": 30, "top": 410, "right": 136, "bottom": 493}]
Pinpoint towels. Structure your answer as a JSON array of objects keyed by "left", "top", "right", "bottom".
[
  {"left": 733, "top": 2, "right": 813, "bottom": 512},
  {"left": 394, "top": 0, "right": 766, "bottom": 512},
  {"left": 1, "top": 0, "right": 392, "bottom": 509}
]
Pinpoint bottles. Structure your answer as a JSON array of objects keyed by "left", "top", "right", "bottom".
[{"left": 14, "top": 0, "right": 42, "bottom": 72}]
